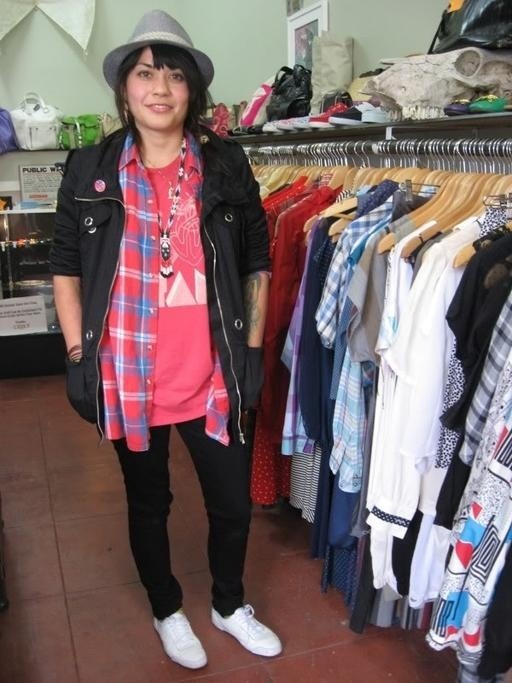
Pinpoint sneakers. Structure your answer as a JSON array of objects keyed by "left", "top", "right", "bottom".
[
  {"left": 262, "top": 102, "right": 389, "bottom": 136},
  {"left": 210, "top": 604, "right": 283, "bottom": 658},
  {"left": 152, "top": 608, "right": 207, "bottom": 670}
]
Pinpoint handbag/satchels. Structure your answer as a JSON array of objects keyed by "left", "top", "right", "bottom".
[
  {"left": 212, "top": 78, "right": 274, "bottom": 141},
  {"left": 426, "top": 0, "right": 512, "bottom": 55},
  {"left": 0, "top": 90, "right": 123, "bottom": 155}
]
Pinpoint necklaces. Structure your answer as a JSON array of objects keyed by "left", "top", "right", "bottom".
[{"left": 143, "top": 148, "right": 182, "bottom": 201}]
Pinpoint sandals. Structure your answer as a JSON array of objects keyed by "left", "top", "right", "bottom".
[{"left": 441, "top": 88, "right": 512, "bottom": 115}]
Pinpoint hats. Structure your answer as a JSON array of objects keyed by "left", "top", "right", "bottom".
[{"left": 102, "top": 9, "right": 215, "bottom": 96}]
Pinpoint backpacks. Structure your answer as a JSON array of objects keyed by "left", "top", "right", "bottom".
[{"left": 270, "top": 64, "right": 311, "bottom": 121}]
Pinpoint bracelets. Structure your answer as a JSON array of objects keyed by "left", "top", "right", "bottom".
[{"left": 62, "top": 344, "right": 84, "bottom": 365}]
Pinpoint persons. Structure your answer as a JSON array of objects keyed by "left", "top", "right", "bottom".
[{"left": 46, "top": 10, "right": 286, "bottom": 671}]
